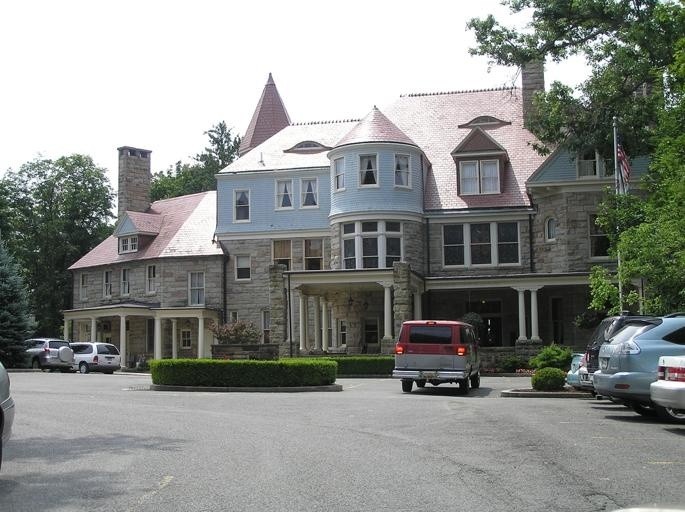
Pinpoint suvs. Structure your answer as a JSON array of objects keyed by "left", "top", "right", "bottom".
[
  {"left": 17, "top": 337, "right": 122, "bottom": 374},
  {"left": 566, "top": 310, "right": 685, "bottom": 425}
]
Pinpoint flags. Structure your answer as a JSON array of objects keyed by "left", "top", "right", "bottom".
[{"left": 617, "top": 125, "right": 632, "bottom": 197}]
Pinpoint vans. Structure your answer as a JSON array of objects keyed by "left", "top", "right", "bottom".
[{"left": 391, "top": 319, "right": 481, "bottom": 394}]
